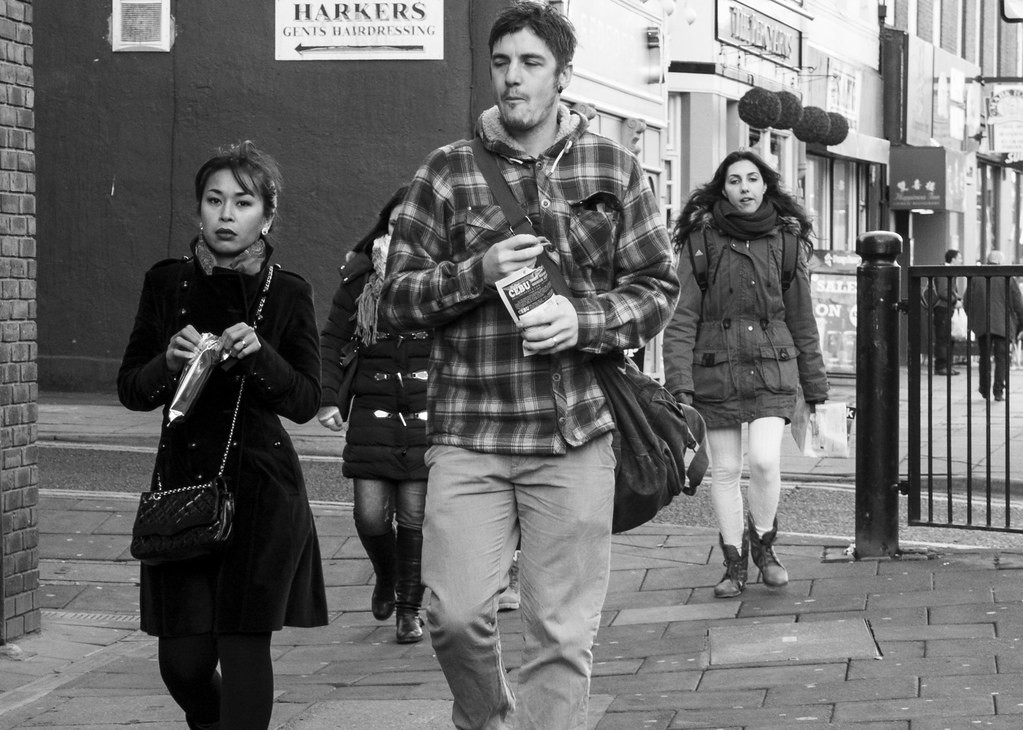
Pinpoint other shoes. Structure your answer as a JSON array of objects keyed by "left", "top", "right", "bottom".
[
  {"left": 995, "top": 394, "right": 1005, "bottom": 400},
  {"left": 934, "top": 367, "right": 959, "bottom": 375},
  {"left": 978, "top": 387, "right": 988, "bottom": 397}
]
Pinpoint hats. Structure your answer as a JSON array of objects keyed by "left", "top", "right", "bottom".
[{"left": 987, "top": 251, "right": 1004, "bottom": 263}]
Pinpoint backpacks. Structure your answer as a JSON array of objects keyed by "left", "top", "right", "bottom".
[{"left": 920, "top": 278, "right": 940, "bottom": 311}]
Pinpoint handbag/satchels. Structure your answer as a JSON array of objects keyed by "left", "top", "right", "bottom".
[
  {"left": 677, "top": 399, "right": 710, "bottom": 495},
  {"left": 593, "top": 348, "right": 686, "bottom": 534},
  {"left": 130, "top": 474, "right": 233, "bottom": 566}
]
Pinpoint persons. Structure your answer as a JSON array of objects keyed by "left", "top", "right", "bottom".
[
  {"left": 117, "top": 149, "right": 328, "bottom": 730},
  {"left": 496, "top": 535, "right": 520, "bottom": 611},
  {"left": 319, "top": 185, "right": 428, "bottom": 642},
  {"left": 376, "top": 0, "right": 681, "bottom": 730},
  {"left": 932, "top": 250, "right": 963, "bottom": 375},
  {"left": 663, "top": 151, "right": 829, "bottom": 598},
  {"left": 963, "top": 252, "right": 1023, "bottom": 400}
]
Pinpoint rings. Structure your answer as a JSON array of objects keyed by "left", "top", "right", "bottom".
[
  {"left": 240, "top": 339, "right": 247, "bottom": 348},
  {"left": 552, "top": 337, "right": 558, "bottom": 348}
]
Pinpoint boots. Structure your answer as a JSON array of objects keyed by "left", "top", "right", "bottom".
[
  {"left": 747, "top": 511, "right": 790, "bottom": 586},
  {"left": 499, "top": 548, "right": 521, "bottom": 608},
  {"left": 714, "top": 527, "right": 749, "bottom": 598},
  {"left": 394, "top": 522, "right": 426, "bottom": 643},
  {"left": 352, "top": 510, "right": 398, "bottom": 620}
]
